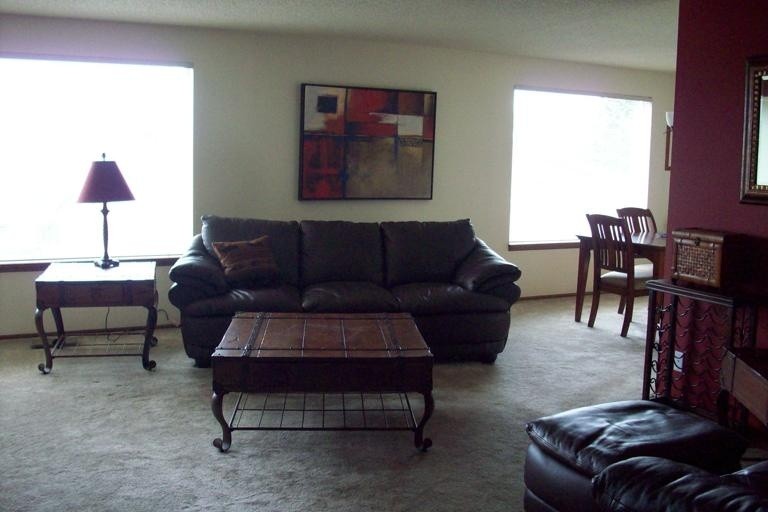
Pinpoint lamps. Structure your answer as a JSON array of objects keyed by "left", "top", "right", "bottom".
[{"left": 78, "top": 159, "right": 136, "bottom": 267}]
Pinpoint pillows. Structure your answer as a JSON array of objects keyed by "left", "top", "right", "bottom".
[{"left": 212, "top": 235, "right": 284, "bottom": 288}]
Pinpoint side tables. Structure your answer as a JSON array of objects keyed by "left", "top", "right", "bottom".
[{"left": 33, "top": 260, "right": 158, "bottom": 374}]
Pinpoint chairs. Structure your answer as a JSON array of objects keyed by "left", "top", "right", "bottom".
[
  {"left": 587, "top": 213, "right": 657, "bottom": 339},
  {"left": 617, "top": 204, "right": 659, "bottom": 315}
]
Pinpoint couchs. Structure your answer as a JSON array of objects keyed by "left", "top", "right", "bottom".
[
  {"left": 168, "top": 214, "right": 524, "bottom": 364},
  {"left": 522, "top": 398, "right": 749, "bottom": 509},
  {"left": 584, "top": 444, "right": 768, "bottom": 510}
]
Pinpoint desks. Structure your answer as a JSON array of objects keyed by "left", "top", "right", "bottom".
[{"left": 576, "top": 231, "right": 665, "bottom": 336}]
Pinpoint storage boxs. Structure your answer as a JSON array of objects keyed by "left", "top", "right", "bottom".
[{"left": 669, "top": 226, "right": 768, "bottom": 298}]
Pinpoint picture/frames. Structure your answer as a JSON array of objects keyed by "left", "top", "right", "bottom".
[
  {"left": 297, "top": 81, "right": 438, "bottom": 202},
  {"left": 662, "top": 124, "right": 675, "bottom": 172}
]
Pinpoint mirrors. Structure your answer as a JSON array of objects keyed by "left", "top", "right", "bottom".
[{"left": 738, "top": 54, "right": 767, "bottom": 209}]
